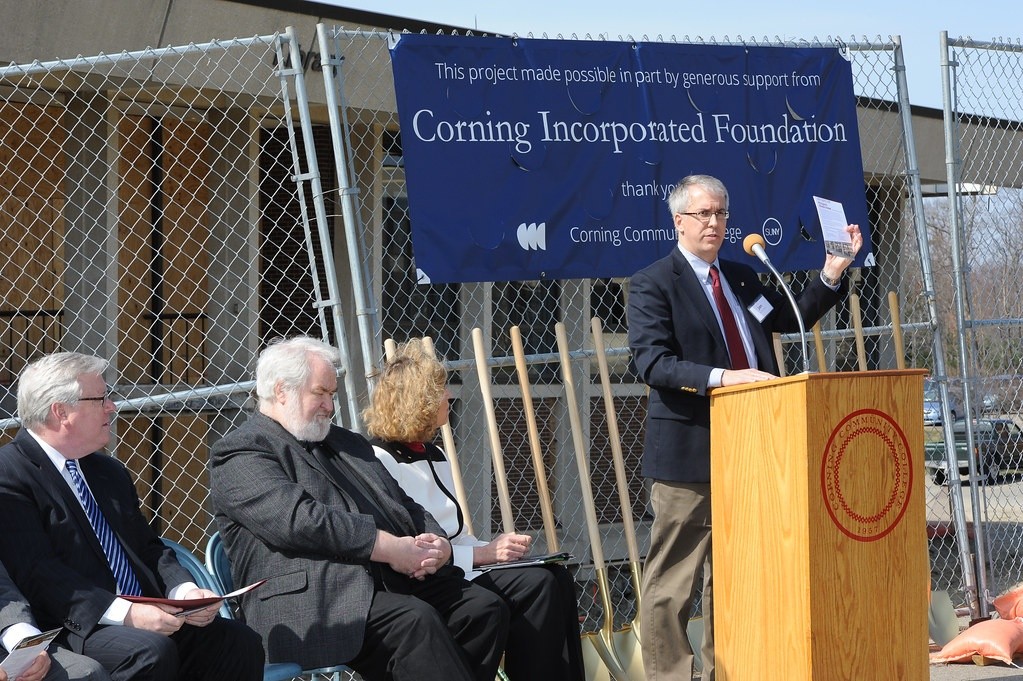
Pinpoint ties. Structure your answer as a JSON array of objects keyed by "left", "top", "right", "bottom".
[
  {"left": 65, "top": 459, "right": 143, "bottom": 598},
  {"left": 709, "top": 266, "right": 750, "bottom": 370},
  {"left": 311, "top": 447, "right": 400, "bottom": 536}
]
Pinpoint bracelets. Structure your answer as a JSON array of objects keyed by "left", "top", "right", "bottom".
[{"left": 821, "top": 269, "right": 841, "bottom": 286}]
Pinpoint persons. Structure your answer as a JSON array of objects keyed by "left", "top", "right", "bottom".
[
  {"left": 0, "top": 560, "right": 114, "bottom": 681},
  {"left": 363, "top": 339, "right": 578, "bottom": 681},
  {"left": 210, "top": 336, "right": 510, "bottom": 681},
  {"left": 628, "top": 175, "right": 862, "bottom": 681},
  {"left": 0, "top": 352, "right": 266, "bottom": 681}
]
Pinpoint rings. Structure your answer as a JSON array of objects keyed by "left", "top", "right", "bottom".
[
  {"left": 207, "top": 616, "right": 210, "bottom": 621},
  {"left": 42, "top": 676, "right": 44, "bottom": 680}
]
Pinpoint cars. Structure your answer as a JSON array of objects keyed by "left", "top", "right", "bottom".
[
  {"left": 924, "top": 374, "right": 1023, "bottom": 414},
  {"left": 924, "top": 390, "right": 978, "bottom": 427}
]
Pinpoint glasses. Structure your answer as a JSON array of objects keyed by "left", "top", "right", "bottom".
[
  {"left": 679, "top": 209, "right": 729, "bottom": 220},
  {"left": 78, "top": 392, "right": 107, "bottom": 407}
]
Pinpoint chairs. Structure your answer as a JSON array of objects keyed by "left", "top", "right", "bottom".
[{"left": 157, "top": 530, "right": 350, "bottom": 681}]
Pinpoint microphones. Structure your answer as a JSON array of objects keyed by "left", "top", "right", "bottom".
[{"left": 743, "top": 233, "right": 817, "bottom": 375}]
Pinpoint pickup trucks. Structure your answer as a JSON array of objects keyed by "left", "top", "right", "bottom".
[{"left": 924, "top": 418, "right": 1023, "bottom": 486}]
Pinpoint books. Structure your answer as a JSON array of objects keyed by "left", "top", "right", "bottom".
[{"left": 473, "top": 553, "right": 575, "bottom": 571}]
